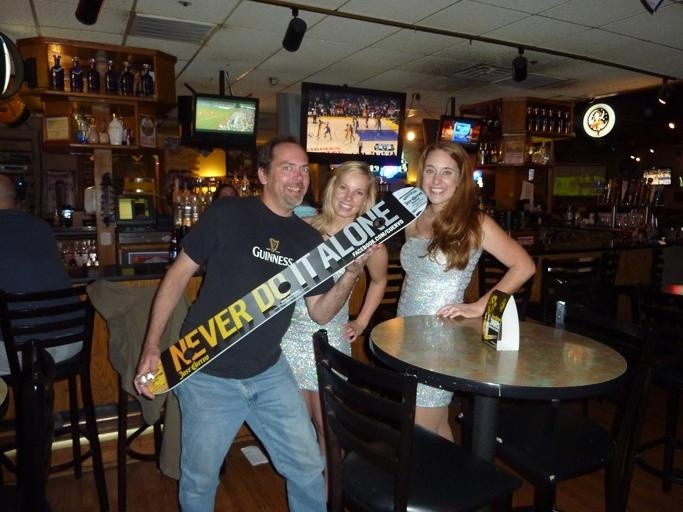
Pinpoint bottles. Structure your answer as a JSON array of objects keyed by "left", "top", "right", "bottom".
[
  {"left": 615, "top": 209, "right": 643, "bottom": 229},
  {"left": 600, "top": 177, "right": 663, "bottom": 205},
  {"left": 478, "top": 142, "right": 504, "bottom": 165},
  {"left": 527, "top": 107, "right": 570, "bottom": 133},
  {"left": 565, "top": 204, "right": 595, "bottom": 226},
  {"left": 169, "top": 171, "right": 251, "bottom": 262},
  {"left": 51, "top": 54, "right": 153, "bottom": 145},
  {"left": 483, "top": 199, "right": 496, "bottom": 219},
  {"left": 62, "top": 240, "right": 97, "bottom": 266}
]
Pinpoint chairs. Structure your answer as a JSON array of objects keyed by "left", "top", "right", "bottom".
[
  {"left": 88, "top": 280, "right": 225, "bottom": 512},
  {"left": 540, "top": 253, "right": 619, "bottom": 328},
  {"left": 632, "top": 285, "right": 683, "bottom": 494},
  {"left": 365, "top": 260, "right": 405, "bottom": 333},
  {"left": 479, "top": 255, "right": 538, "bottom": 321},
  {"left": 0, "top": 280, "right": 109, "bottom": 512},
  {"left": 456, "top": 268, "right": 663, "bottom": 512},
  {"left": 312, "top": 329, "right": 523, "bottom": 512}
]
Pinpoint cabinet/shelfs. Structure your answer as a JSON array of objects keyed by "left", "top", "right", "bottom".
[
  {"left": 0, "top": 36, "right": 177, "bottom": 267},
  {"left": 459, "top": 96, "right": 576, "bottom": 214}
]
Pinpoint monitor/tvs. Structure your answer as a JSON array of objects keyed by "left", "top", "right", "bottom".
[
  {"left": 299, "top": 81, "right": 406, "bottom": 166},
  {"left": 437, "top": 114, "right": 484, "bottom": 154},
  {"left": 545, "top": 161, "right": 611, "bottom": 201},
  {"left": 115, "top": 194, "right": 157, "bottom": 231},
  {"left": 641, "top": 167, "right": 673, "bottom": 187},
  {"left": 177, "top": 95, "right": 193, "bottom": 148},
  {"left": 191, "top": 92, "right": 260, "bottom": 152},
  {"left": 422, "top": 118, "right": 440, "bottom": 148}
]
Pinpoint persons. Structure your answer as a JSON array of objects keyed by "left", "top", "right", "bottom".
[
  {"left": 212, "top": 184, "right": 238, "bottom": 200},
  {"left": 0, "top": 174, "right": 86, "bottom": 378},
  {"left": 309, "top": 92, "right": 401, "bottom": 155},
  {"left": 393, "top": 143, "right": 537, "bottom": 442},
  {"left": 280, "top": 162, "right": 389, "bottom": 476},
  {"left": 133, "top": 138, "right": 384, "bottom": 512}
]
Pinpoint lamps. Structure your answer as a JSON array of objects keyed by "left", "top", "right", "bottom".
[
  {"left": 75, "top": 0, "right": 104, "bottom": 25},
  {"left": 512, "top": 49, "right": 528, "bottom": 82},
  {"left": 282, "top": 8, "right": 307, "bottom": 52}
]
